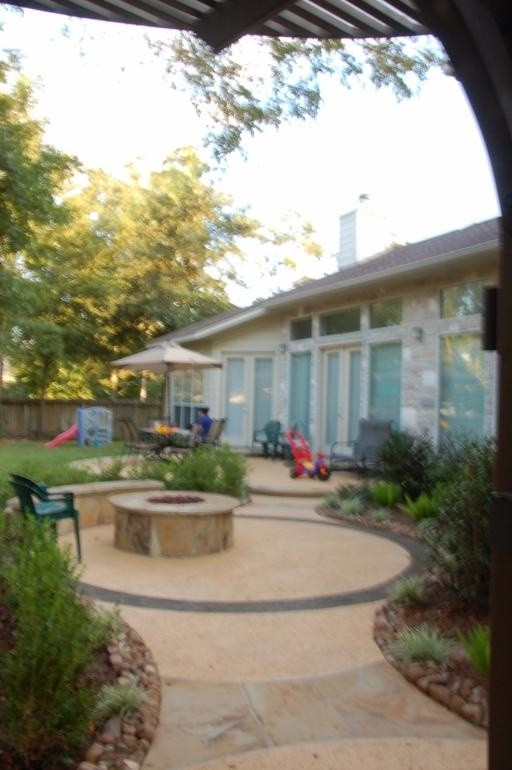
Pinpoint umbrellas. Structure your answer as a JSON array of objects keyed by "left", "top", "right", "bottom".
[{"left": 108, "top": 338, "right": 225, "bottom": 425}]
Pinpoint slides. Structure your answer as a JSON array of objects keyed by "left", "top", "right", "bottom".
[{"left": 44, "top": 424, "right": 77, "bottom": 446}]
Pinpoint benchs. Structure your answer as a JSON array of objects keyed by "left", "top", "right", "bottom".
[{"left": 326, "top": 416, "right": 395, "bottom": 477}]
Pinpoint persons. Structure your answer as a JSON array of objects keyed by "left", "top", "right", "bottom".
[{"left": 134, "top": 406, "right": 214, "bottom": 451}]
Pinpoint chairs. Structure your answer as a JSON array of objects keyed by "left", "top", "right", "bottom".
[
  {"left": 115, "top": 415, "right": 229, "bottom": 467},
  {"left": 249, "top": 418, "right": 298, "bottom": 460},
  {"left": 8, "top": 472, "right": 82, "bottom": 563}
]
